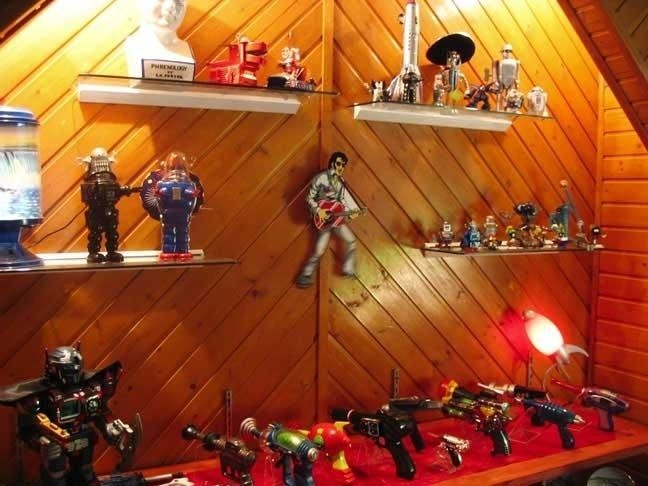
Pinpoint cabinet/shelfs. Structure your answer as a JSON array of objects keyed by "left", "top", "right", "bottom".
[
  {"left": 71, "top": 61, "right": 343, "bottom": 118},
  {"left": 347, "top": 90, "right": 558, "bottom": 140},
  {"left": 417, "top": 228, "right": 610, "bottom": 271},
  {"left": 1, "top": 244, "right": 243, "bottom": 285}
]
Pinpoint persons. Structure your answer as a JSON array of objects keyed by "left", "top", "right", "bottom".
[
  {"left": 432, "top": 179, "right": 605, "bottom": 252},
  {"left": 81, "top": 145, "right": 143, "bottom": 262},
  {"left": 2, "top": 341, "right": 134, "bottom": 485},
  {"left": 125, "top": 0, "right": 196, "bottom": 85},
  {"left": 137, "top": 150, "right": 206, "bottom": 259},
  {"left": 362, "top": 0, "right": 549, "bottom": 118},
  {"left": 292, "top": 151, "right": 363, "bottom": 287}
]
